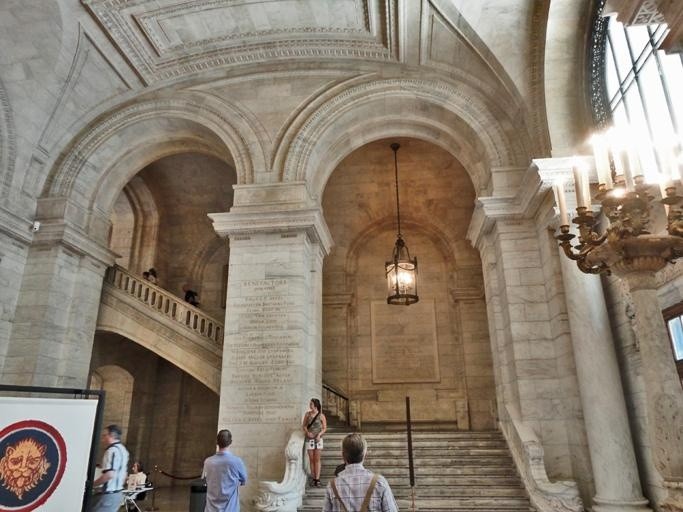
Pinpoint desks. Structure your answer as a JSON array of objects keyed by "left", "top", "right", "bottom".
[{"left": 91, "top": 486, "right": 154, "bottom": 512}]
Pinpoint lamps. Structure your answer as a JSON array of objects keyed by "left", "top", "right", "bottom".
[
  {"left": 550, "top": 140, "right": 683, "bottom": 273},
  {"left": 384, "top": 144, "right": 418, "bottom": 306}
]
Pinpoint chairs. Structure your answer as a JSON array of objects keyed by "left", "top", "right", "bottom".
[{"left": 134, "top": 473, "right": 150, "bottom": 500}]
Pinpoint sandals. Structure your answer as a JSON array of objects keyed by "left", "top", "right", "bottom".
[{"left": 310, "top": 478, "right": 321, "bottom": 487}]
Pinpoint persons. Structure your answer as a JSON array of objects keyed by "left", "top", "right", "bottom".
[
  {"left": 148, "top": 267, "right": 156, "bottom": 283},
  {"left": 302, "top": 398, "right": 327, "bottom": 487},
  {"left": 201, "top": 429, "right": 248, "bottom": 512},
  {"left": 126, "top": 460, "right": 147, "bottom": 512},
  {"left": 143, "top": 271, "right": 149, "bottom": 280},
  {"left": 322, "top": 433, "right": 400, "bottom": 512},
  {"left": 182, "top": 283, "right": 198, "bottom": 307},
  {"left": 91, "top": 424, "right": 130, "bottom": 512}
]
